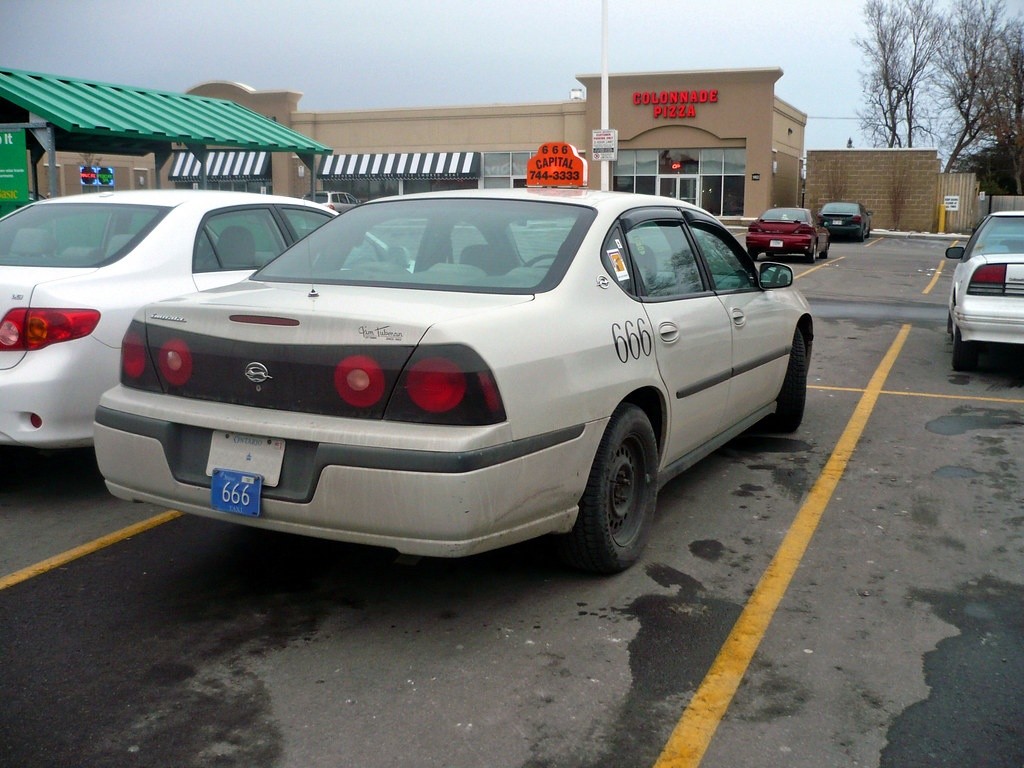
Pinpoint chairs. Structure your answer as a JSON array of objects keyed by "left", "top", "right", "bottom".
[
  {"left": 215, "top": 226, "right": 255, "bottom": 267},
  {"left": 459, "top": 243, "right": 512, "bottom": 274},
  {"left": 9, "top": 227, "right": 56, "bottom": 260},
  {"left": 1000, "top": 240, "right": 1024, "bottom": 253},
  {"left": 105, "top": 233, "right": 134, "bottom": 256}
]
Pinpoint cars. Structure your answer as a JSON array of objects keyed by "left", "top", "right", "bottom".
[
  {"left": 300, "top": 191, "right": 362, "bottom": 216},
  {"left": 944, "top": 211, "right": 1024, "bottom": 370},
  {"left": 0, "top": 188, "right": 417, "bottom": 449},
  {"left": 745, "top": 206, "right": 831, "bottom": 263},
  {"left": 97, "top": 141, "right": 817, "bottom": 578},
  {"left": 818, "top": 202, "right": 874, "bottom": 242}
]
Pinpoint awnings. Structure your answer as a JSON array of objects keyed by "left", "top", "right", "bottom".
[
  {"left": 316, "top": 152, "right": 480, "bottom": 182},
  {"left": 168, "top": 149, "right": 272, "bottom": 183}
]
